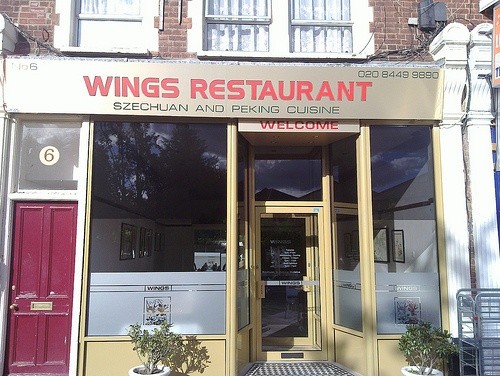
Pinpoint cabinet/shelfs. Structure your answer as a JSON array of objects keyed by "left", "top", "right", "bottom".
[{"left": 455, "top": 288, "right": 500, "bottom": 376}]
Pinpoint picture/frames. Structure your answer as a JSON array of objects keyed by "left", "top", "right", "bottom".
[
  {"left": 160, "top": 233, "right": 165, "bottom": 251},
  {"left": 139, "top": 227, "right": 144, "bottom": 258},
  {"left": 344, "top": 233, "right": 352, "bottom": 255},
  {"left": 374, "top": 228, "right": 390, "bottom": 263},
  {"left": 391, "top": 230, "right": 405, "bottom": 263},
  {"left": 155, "top": 232, "right": 161, "bottom": 251},
  {"left": 120, "top": 222, "right": 137, "bottom": 261},
  {"left": 352, "top": 230, "right": 359, "bottom": 255},
  {"left": 148, "top": 229, "right": 152, "bottom": 256}
]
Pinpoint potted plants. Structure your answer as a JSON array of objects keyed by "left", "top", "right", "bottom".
[
  {"left": 125, "top": 322, "right": 182, "bottom": 376},
  {"left": 397, "top": 322, "right": 461, "bottom": 376}
]
